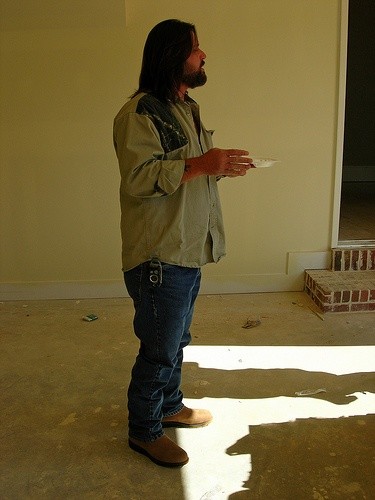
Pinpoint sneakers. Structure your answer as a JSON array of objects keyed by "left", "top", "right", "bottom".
[
  {"left": 161, "top": 405, "right": 213, "bottom": 429},
  {"left": 128, "top": 432, "right": 189, "bottom": 467}
]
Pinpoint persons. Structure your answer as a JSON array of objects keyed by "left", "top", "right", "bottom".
[{"left": 110, "top": 18, "right": 259, "bottom": 470}]
